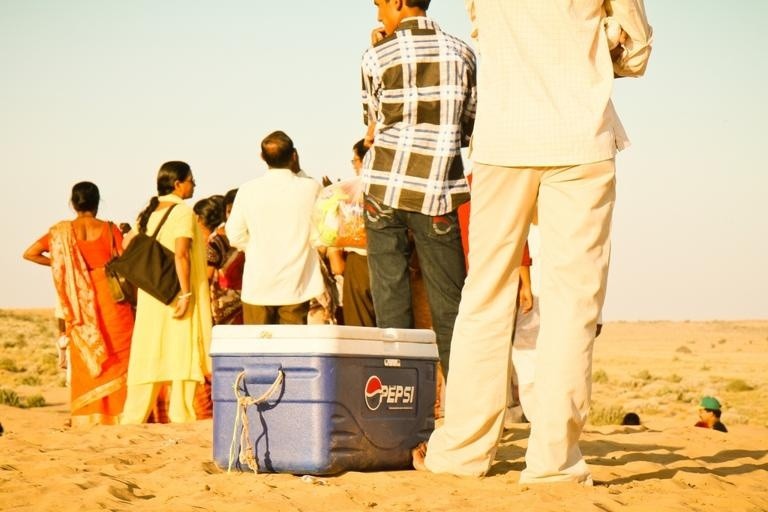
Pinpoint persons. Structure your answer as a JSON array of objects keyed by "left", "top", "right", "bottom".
[
  {"left": 322, "top": 138, "right": 541, "bottom": 430},
  {"left": 226, "top": 131, "right": 325, "bottom": 323},
  {"left": 120, "top": 161, "right": 215, "bottom": 426},
  {"left": 622, "top": 413, "right": 642, "bottom": 427},
  {"left": 361, "top": 0, "right": 470, "bottom": 382},
  {"left": 409, "top": 0, "right": 661, "bottom": 486},
  {"left": 692, "top": 395, "right": 730, "bottom": 434},
  {"left": 23, "top": 182, "right": 137, "bottom": 430},
  {"left": 143, "top": 189, "right": 244, "bottom": 423}
]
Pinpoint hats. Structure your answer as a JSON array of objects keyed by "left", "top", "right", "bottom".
[{"left": 697, "top": 396, "right": 721, "bottom": 410}]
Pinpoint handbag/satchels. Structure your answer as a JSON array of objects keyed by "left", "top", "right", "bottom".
[{"left": 104, "top": 232, "right": 181, "bottom": 306}]
